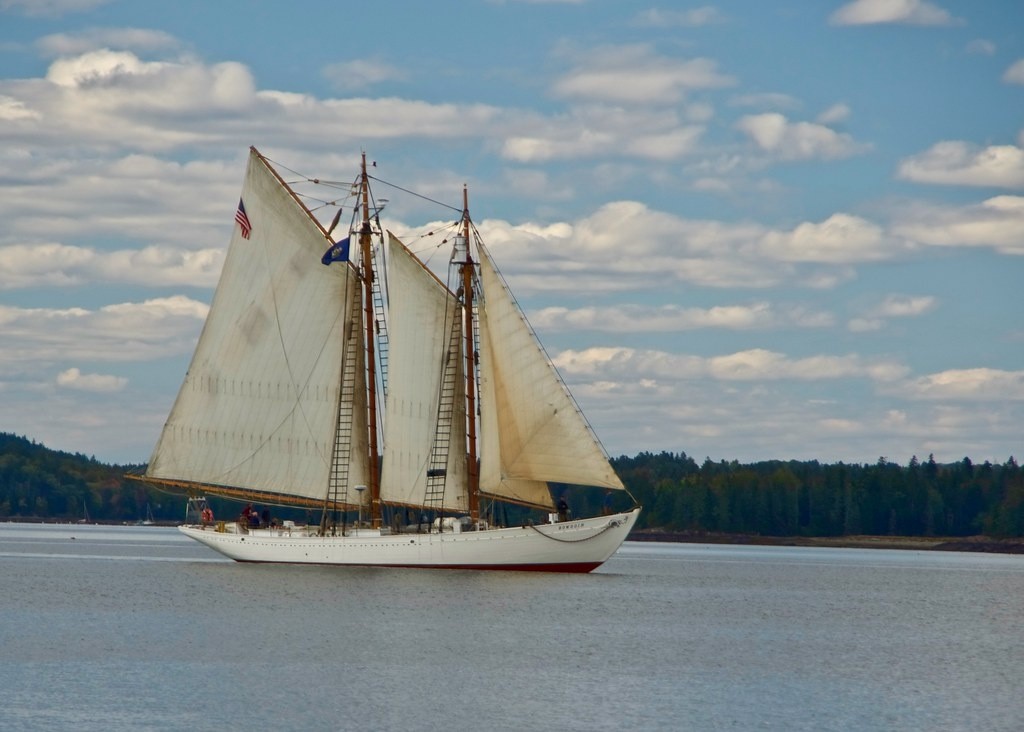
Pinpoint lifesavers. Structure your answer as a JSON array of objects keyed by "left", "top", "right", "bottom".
[{"left": 202, "top": 508, "right": 213, "bottom": 521}]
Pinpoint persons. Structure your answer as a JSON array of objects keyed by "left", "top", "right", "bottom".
[
  {"left": 241, "top": 502, "right": 272, "bottom": 529},
  {"left": 556, "top": 494, "right": 569, "bottom": 523},
  {"left": 603, "top": 487, "right": 613, "bottom": 515}
]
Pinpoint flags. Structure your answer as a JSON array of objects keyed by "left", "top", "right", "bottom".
[
  {"left": 320, "top": 238, "right": 350, "bottom": 266},
  {"left": 234, "top": 196, "right": 252, "bottom": 241}
]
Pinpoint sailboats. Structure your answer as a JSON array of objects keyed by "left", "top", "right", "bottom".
[
  {"left": 77, "top": 500, "right": 88, "bottom": 524},
  {"left": 122, "top": 144, "right": 643, "bottom": 573}
]
